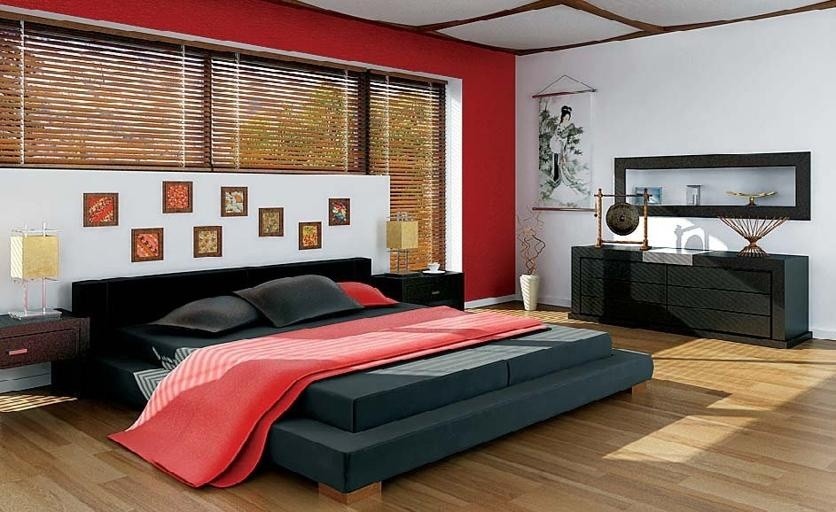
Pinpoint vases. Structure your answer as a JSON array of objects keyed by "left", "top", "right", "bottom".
[{"left": 519, "top": 274, "right": 540, "bottom": 312}]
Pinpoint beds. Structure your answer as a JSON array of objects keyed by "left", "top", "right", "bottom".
[{"left": 58, "top": 259, "right": 654, "bottom": 505}]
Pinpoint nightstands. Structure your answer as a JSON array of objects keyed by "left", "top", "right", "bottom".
[
  {"left": 0, "top": 308, "right": 91, "bottom": 392},
  {"left": 371, "top": 268, "right": 465, "bottom": 313}
]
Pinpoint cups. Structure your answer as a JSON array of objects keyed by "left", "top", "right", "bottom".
[{"left": 428, "top": 264, "right": 441, "bottom": 272}]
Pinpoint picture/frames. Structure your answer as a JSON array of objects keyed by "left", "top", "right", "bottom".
[
  {"left": 329, "top": 198, "right": 351, "bottom": 226},
  {"left": 298, "top": 221, "right": 322, "bottom": 250},
  {"left": 193, "top": 226, "right": 222, "bottom": 257},
  {"left": 82, "top": 192, "right": 120, "bottom": 228},
  {"left": 130, "top": 228, "right": 165, "bottom": 263},
  {"left": 258, "top": 207, "right": 285, "bottom": 238},
  {"left": 220, "top": 186, "right": 249, "bottom": 217},
  {"left": 161, "top": 180, "right": 193, "bottom": 214}
]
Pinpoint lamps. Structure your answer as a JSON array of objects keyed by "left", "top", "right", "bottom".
[
  {"left": 8, "top": 221, "right": 61, "bottom": 319},
  {"left": 385, "top": 211, "right": 422, "bottom": 276}
]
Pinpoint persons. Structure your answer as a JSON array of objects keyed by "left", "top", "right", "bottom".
[{"left": 543, "top": 104, "right": 586, "bottom": 201}]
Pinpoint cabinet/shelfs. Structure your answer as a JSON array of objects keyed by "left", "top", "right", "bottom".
[
  {"left": 568, "top": 244, "right": 812, "bottom": 350},
  {"left": 614, "top": 151, "right": 811, "bottom": 222}
]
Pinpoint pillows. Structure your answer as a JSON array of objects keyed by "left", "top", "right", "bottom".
[
  {"left": 336, "top": 280, "right": 400, "bottom": 308},
  {"left": 147, "top": 294, "right": 260, "bottom": 334},
  {"left": 236, "top": 274, "right": 365, "bottom": 328}
]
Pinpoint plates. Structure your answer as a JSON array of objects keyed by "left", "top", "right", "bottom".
[{"left": 422, "top": 270, "right": 445, "bottom": 275}]
